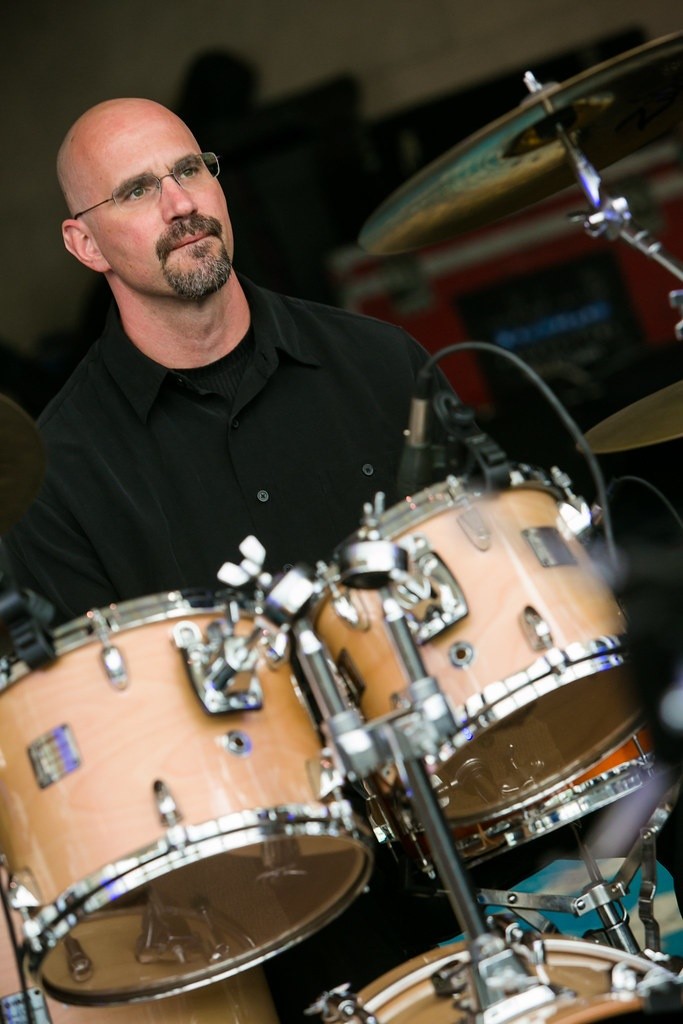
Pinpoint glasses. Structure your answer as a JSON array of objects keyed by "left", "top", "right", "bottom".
[{"left": 74, "top": 151, "right": 220, "bottom": 220}]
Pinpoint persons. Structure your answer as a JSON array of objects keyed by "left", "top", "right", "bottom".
[{"left": 0, "top": 97, "right": 559, "bottom": 1024}]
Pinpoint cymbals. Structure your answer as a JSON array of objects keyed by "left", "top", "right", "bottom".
[
  {"left": 571, "top": 373, "right": 681, "bottom": 456},
  {"left": 356, "top": 28, "right": 681, "bottom": 255}
]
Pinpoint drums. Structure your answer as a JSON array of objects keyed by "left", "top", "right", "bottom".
[{"left": 0, "top": 462, "right": 683, "bottom": 1022}]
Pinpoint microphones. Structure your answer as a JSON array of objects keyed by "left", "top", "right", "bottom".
[{"left": 394, "top": 367, "right": 432, "bottom": 501}]
